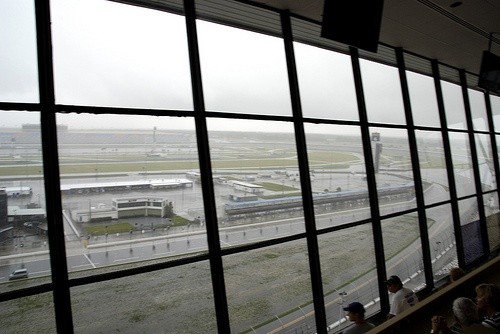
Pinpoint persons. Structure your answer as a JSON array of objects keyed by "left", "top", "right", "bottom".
[
  {"left": 383, "top": 275, "right": 420, "bottom": 320},
  {"left": 342, "top": 302, "right": 375, "bottom": 334},
  {"left": 449, "top": 266, "right": 466, "bottom": 282},
  {"left": 431, "top": 283, "right": 500, "bottom": 334}
]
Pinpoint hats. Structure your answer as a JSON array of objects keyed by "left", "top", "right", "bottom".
[
  {"left": 343, "top": 302, "right": 364, "bottom": 312},
  {"left": 384, "top": 276, "right": 402, "bottom": 285}
]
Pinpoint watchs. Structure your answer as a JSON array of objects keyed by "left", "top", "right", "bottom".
[{"left": 430, "top": 329, "right": 437, "bottom": 334}]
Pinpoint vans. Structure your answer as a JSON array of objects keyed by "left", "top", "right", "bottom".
[{"left": 9, "top": 269, "right": 28, "bottom": 280}]
